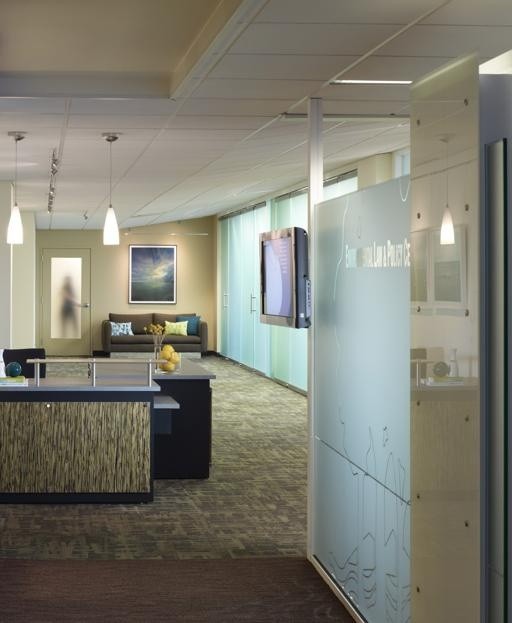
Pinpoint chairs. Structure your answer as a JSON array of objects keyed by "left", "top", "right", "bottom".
[{"left": 3, "top": 348, "right": 46, "bottom": 378}]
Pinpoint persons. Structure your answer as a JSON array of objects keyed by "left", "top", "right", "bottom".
[{"left": 59, "top": 274, "right": 83, "bottom": 338}]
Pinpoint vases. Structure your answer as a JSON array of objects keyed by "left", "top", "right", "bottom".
[{"left": 155, "top": 346, "right": 162, "bottom": 370}]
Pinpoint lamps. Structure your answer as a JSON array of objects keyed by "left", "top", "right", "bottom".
[
  {"left": 5, "top": 130, "right": 24, "bottom": 244},
  {"left": 101, "top": 131, "right": 122, "bottom": 246}
]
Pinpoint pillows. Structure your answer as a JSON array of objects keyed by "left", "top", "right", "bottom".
[
  {"left": 109, "top": 321, "right": 135, "bottom": 336},
  {"left": 163, "top": 316, "right": 201, "bottom": 336}
]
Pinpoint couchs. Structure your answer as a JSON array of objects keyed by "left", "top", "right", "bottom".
[{"left": 101, "top": 312, "right": 208, "bottom": 360}]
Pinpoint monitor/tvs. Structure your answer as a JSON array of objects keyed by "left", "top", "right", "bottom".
[{"left": 259, "top": 227, "right": 312, "bottom": 329}]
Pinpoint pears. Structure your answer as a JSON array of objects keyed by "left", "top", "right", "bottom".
[{"left": 159, "top": 344, "right": 179, "bottom": 370}]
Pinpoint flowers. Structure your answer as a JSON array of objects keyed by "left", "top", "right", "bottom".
[{"left": 143, "top": 321, "right": 167, "bottom": 346}]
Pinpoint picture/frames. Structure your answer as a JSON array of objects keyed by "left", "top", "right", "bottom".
[{"left": 127, "top": 244, "right": 178, "bottom": 305}]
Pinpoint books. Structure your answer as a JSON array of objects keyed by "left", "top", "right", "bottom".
[
  {"left": 0, "top": 375, "right": 26, "bottom": 384},
  {"left": 0, "top": 378, "right": 30, "bottom": 388},
  {"left": 418, "top": 374, "right": 465, "bottom": 388}
]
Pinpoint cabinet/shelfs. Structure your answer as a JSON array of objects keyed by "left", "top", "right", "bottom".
[{"left": 150, "top": 359, "right": 216, "bottom": 480}]
